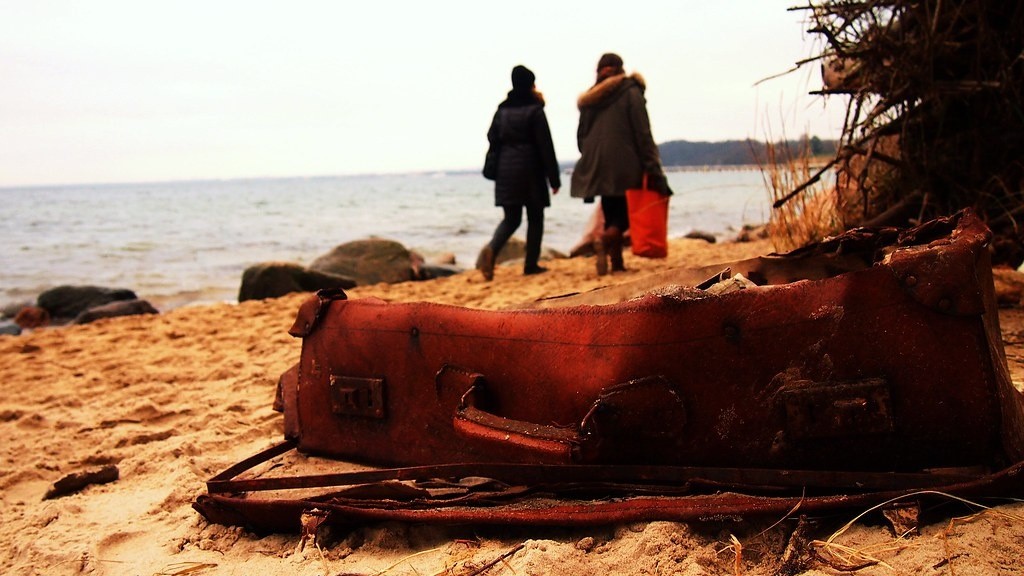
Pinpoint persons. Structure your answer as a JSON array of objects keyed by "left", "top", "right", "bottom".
[
  {"left": 475, "top": 65, "right": 561, "bottom": 282},
  {"left": 570, "top": 54, "right": 673, "bottom": 277}
]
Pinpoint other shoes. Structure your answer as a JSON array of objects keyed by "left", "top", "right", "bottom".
[
  {"left": 476, "top": 245, "right": 493, "bottom": 280},
  {"left": 525, "top": 262, "right": 548, "bottom": 274}
]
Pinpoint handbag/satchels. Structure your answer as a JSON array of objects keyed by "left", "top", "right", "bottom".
[
  {"left": 192, "top": 208, "right": 1024, "bottom": 526},
  {"left": 626, "top": 172, "right": 668, "bottom": 258},
  {"left": 483, "top": 127, "right": 500, "bottom": 180}
]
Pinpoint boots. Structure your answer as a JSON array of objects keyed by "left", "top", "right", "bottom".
[
  {"left": 609, "top": 238, "right": 627, "bottom": 271},
  {"left": 595, "top": 228, "right": 620, "bottom": 276}
]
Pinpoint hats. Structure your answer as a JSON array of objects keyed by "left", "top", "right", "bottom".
[
  {"left": 597, "top": 54, "right": 623, "bottom": 72},
  {"left": 512, "top": 65, "right": 535, "bottom": 89}
]
